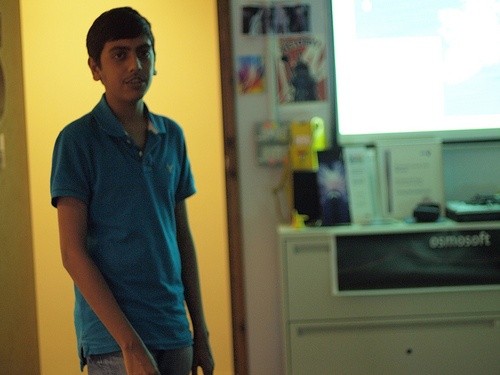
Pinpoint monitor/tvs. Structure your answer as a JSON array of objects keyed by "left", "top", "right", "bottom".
[{"left": 331, "top": 0, "right": 500, "bottom": 147}]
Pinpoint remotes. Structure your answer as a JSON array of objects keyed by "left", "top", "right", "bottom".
[{"left": 413, "top": 201, "right": 440, "bottom": 221}]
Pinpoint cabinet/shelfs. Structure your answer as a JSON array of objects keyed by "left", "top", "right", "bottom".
[{"left": 277, "top": 215, "right": 500, "bottom": 375}]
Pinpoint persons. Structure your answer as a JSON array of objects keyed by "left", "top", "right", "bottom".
[{"left": 50, "top": 7, "right": 215, "bottom": 375}]
